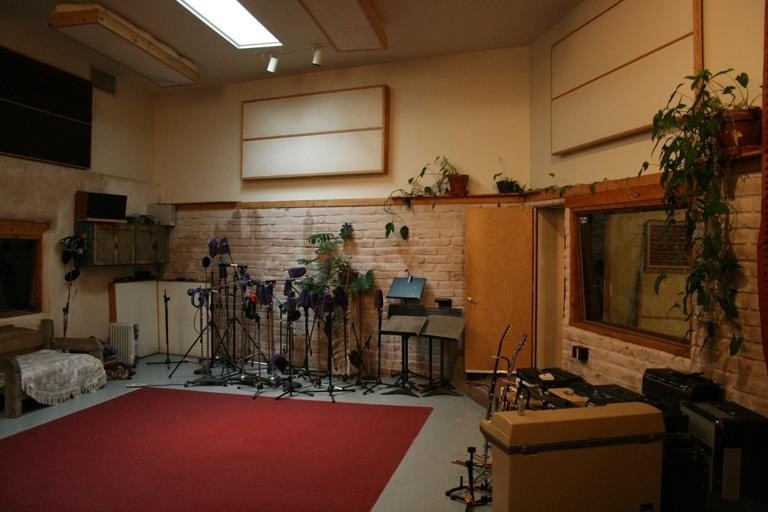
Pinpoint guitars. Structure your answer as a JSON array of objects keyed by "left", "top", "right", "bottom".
[{"left": 495, "top": 333, "right": 528, "bottom": 413}]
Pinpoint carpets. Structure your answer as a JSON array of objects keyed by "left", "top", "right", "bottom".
[{"left": 1, "top": 384, "right": 436, "bottom": 512}]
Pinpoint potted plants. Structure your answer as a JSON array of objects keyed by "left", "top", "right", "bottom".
[
  {"left": 381, "top": 152, "right": 472, "bottom": 241},
  {"left": 636, "top": 66, "right": 763, "bottom": 356}
]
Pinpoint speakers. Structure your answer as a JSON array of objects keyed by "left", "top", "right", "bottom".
[{"left": 76, "top": 191, "right": 127, "bottom": 219}]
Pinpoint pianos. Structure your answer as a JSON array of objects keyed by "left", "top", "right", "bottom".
[{"left": 481, "top": 402, "right": 663, "bottom": 512}]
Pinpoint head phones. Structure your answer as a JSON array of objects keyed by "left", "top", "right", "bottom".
[{"left": 61, "top": 235, "right": 85, "bottom": 282}]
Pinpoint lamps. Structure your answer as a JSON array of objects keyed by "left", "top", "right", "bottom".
[{"left": 260, "top": 44, "right": 327, "bottom": 75}]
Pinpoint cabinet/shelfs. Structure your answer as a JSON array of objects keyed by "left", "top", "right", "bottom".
[{"left": 74, "top": 221, "right": 169, "bottom": 266}]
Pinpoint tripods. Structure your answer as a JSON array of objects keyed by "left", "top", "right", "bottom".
[
  {"left": 168, "top": 238, "right": 356, "bottom": 403},
  {"left": 362, "top": 289, "right": 407, "bottom": 395},
  {"left": 147, "top": 289, "right": 189, "bottom": 369},
  {"left": 342, "top": 323, "right": 378, "bottom": 393},
  {"left": 381, "top": 274, "right": 463, "bottom": 398}
]
